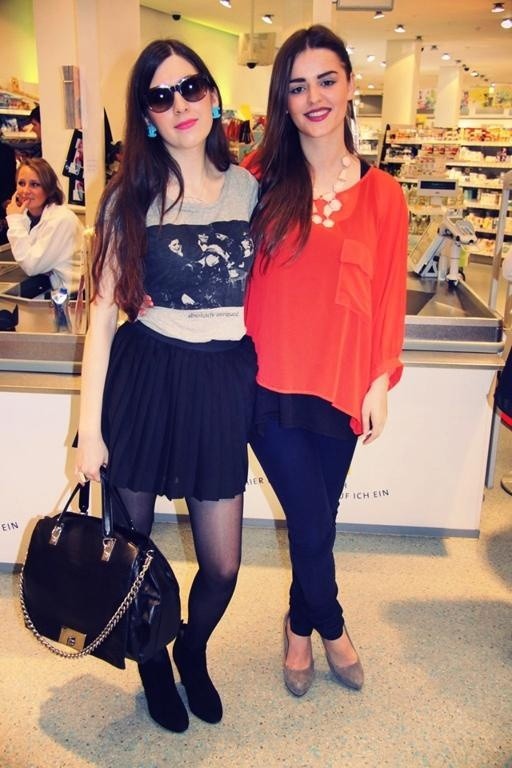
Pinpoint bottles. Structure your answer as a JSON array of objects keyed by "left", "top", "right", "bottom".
[{"left": 53, "top": 288, "right": 72, "bottom": 333}]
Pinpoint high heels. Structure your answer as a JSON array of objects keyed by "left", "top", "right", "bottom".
[
  {"left": 320, "top": 620, "right": 365, "bottom": 690},
  {"left": 135, "top": 639, "right": 190, "bottom": 735},
  {"left": 172, "top": 620, "right": 224, "bottom": 724},
  {"left": 279, "top": 609, "right": 316, "bottom": 697}
]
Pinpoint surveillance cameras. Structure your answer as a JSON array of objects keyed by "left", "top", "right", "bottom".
[
  {"left": 170, "top": 12, "right": 184, "bottom": 23},
  {"left": 246, "top": 61, "right": 258, "bottom": 69},
  {"left": 463, "top": 67, "right": 470, "bottom": 71}
]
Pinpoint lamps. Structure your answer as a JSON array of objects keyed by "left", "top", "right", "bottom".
[
  {"left": 262, "top": 15, "right": 273, "bottom": 24},
  {"left": 395, "top": 25, "right": 406, "bottom": 33},
  {"left": 373, "top": 12, "right": 384, "bottom": 19},
  {"left": 220, "top": 0, "right": 231, "bottom": 9},
  {"left": 491, "top": 4, "right": 503, "bottom": 12},
  {"left": 501, "top": 19, "right": 512, "bottom": 30}
]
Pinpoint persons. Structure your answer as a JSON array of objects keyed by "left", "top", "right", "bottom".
[
  {"left": 7, "top": 154, "right": 86, "bottom": 302},
  {"left": 71, "top": 36, "right": 262, "bottom": 735},
  {"left": 135, "top": 23, "right": 408, "bottom": 697},
  {"left": 28, "top": 105, "right": 41, "bottom": 140}
]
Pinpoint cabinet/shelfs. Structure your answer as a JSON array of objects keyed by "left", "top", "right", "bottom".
[
  {"left": 0, "top": 106, "right": 38, "bottom": 144},
  {"left": 357, "top": 123, "right": 511, "bottom": 266}
]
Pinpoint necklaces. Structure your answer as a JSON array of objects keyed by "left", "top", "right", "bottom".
[{"left": 308, "top": 151, "right": 353, "bottom": 229}]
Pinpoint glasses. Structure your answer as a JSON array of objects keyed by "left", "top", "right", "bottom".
[{"left": 144, "top": 71, "right": 209, "bottom": 114}]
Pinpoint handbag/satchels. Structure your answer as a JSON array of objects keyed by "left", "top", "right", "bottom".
[{"left": 16, "top": 465, "right": 182, "bottom": 671}]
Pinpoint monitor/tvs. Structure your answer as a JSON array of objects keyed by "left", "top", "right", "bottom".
[{"left": 407, "top": 222, "right": 444, "bottom": 274}]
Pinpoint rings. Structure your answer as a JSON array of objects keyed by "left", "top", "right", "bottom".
[{"left": 78, "top": 469, "right": 91, "bottom": 482}]
